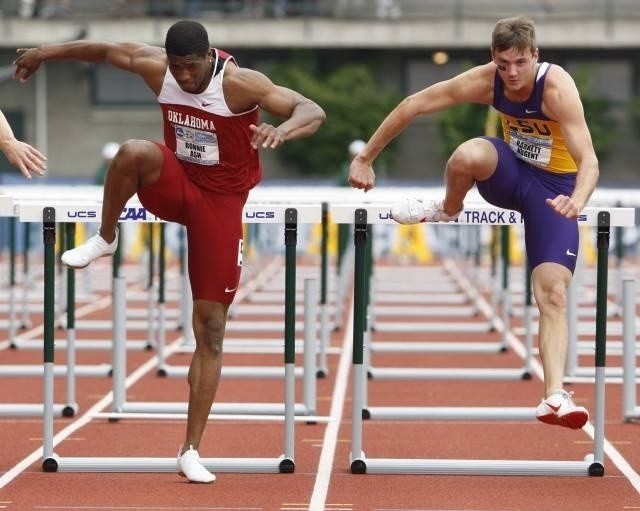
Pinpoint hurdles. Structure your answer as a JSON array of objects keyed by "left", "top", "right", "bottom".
[{"left": 0, "top": 185, "right": 639, "bottom": 477}]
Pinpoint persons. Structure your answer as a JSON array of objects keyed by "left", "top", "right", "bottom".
[
  {"left": 12, "top": 19, "right": 327, "bottom": 484},
  {"left": 0, "top": 108, "right": 49, "bottom": 183},
  {"left": 347, "top": 11, "right": 600, "bottom": 431}
]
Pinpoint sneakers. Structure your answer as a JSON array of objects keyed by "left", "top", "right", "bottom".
[
  {"left": 61, "top": 228, "right": 119, "bottom": 267},
  {"left": 390, "top": 196, "right": 465, "bottom": 223},
  {"left": 176, "top": 446, "right": 215, "bottom": 484},
  {"left": 536, "top": 390, "right": 589, "bottom": 429}
]
